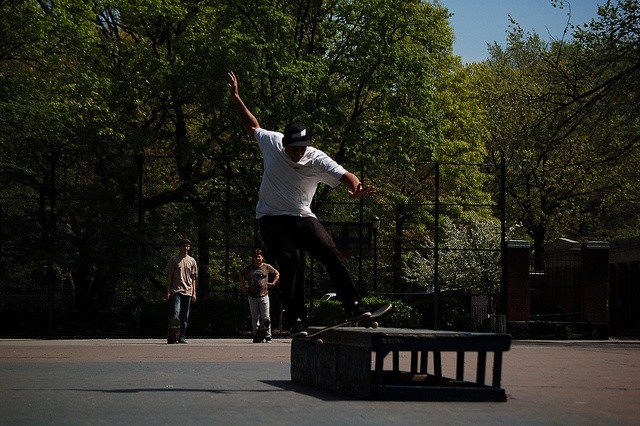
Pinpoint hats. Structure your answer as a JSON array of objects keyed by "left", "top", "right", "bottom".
[
  {"left": 284, "top": 122, "right": 312, "bottom": 147},
  {"left": 179, "top": 239, "right": 192, "bottom": 246}
]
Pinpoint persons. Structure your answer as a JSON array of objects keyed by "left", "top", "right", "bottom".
[
  {"left": 164, "top": 239, "right": 199, "bottom": 343},
  {"left": 239, "top": 248, "right": 280, "bottom": 343},
  {"left": 227, "top": 69, "right": 376, "bottom": 337}
]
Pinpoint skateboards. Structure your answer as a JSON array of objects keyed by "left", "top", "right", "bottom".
[
  {"left": 167, "top": 317, "right": 181, "bottom": 344},
  {"left": 253, "top": 318, "right": 270, "bottom": 343},
  {"left": 290, "top": 303, "right": 392, "bottom": 345}
]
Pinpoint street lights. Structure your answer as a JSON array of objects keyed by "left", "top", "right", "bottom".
[{"left": 370, "top": 214, "right": 380, "bottom": 293}]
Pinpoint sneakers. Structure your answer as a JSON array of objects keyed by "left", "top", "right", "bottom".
[
  {"left": 289, "top": 318, "right": 309, "bottom": 338},
  {"left": 344, "top": 299, "right": 372, "bottom": 321}
]
